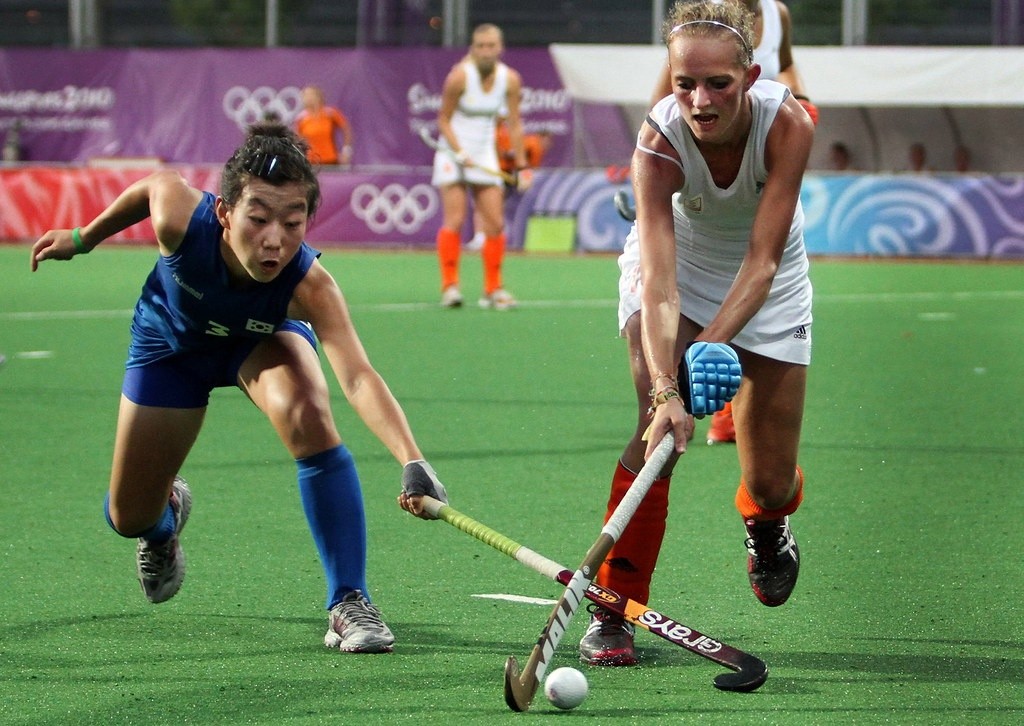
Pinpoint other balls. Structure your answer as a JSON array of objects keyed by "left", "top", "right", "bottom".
[{"left": 543, "top": 666, "right": 590, "bottom": 710}]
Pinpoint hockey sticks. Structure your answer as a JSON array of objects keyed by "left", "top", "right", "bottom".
[
  {"left": 504, "top": 410, "right": 692, "bottom": 713},
  {"left": 419, "top": 126, "right": 515, "bottom": 186},
  {"left": 614, "top": 189, "right": 637, "bottom": 221},
  {"left": 420, "top": 494, "right": 771, "bottom": 693}
]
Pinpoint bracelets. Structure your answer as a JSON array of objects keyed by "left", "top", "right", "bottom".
[
  {"left": 645, "top": 372, "right": 686, "bottom": 414},
  {"left": 72, "top": 224, "right": 90, "bottom": 255}
]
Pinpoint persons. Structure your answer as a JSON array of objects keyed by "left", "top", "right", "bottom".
[
  {"left": 829, "top": 142, "right": 861, "bottom": 173},
  {"left": 30, "top": 108, "right": 451, "bottom": 654},
  {"left": 293, "top": 87, "right": 356, "bottom": 169},
  {"left": 954, "top": 147, "right": 973, "bottom": 170},
  {"left": 430, "top": 24, "right": 544, "bottom": 313},
  {"left": 899, "top": 143, "right": 935, "bottom": 172},
  {"left": 576, "top": 0, "right": 812, "bottom": 665},
  {"left": 646, "top": 0, "right": 820, "bottom": 130}
]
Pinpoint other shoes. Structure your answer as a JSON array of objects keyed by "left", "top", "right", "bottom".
[
  {"left": 708, "top": 402, "right": 736, "bottom": 442},
  {"left": 440, "top": 286, "right": 461, "bottom": 306},
  {"left": 477, "top": 290, "right": 516, "bottom": 309}
]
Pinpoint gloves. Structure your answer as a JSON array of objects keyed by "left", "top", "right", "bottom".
[{"left": 677, "top": 341, "right": 742, "bottom": 419}]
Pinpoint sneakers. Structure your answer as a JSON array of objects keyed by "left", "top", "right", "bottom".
[
  {"left": 579, "top": 603, "right": 637, "bottom": 665},
  {"left": 325, "top": 589, "right": 395, "bottom": 653},
  {"left": 743, "top": 515, "right": 800, "bottom": 608},
  {"left": 135, "top": 474, "right": 193, "bottom": 603}
]
